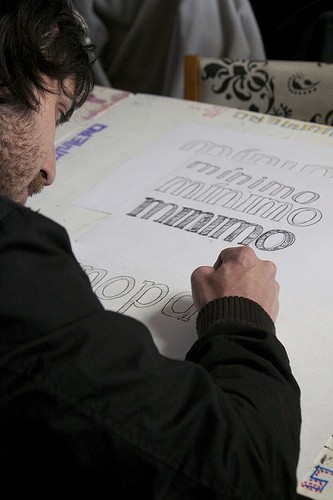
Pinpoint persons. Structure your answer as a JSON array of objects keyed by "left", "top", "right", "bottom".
[{"left": 0, "top": 0, "right": 302, "bottom": 500}]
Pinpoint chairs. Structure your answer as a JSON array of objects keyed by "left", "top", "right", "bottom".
[{"left": 182, "top": 56, "right": 333, "bottom": 126}]
